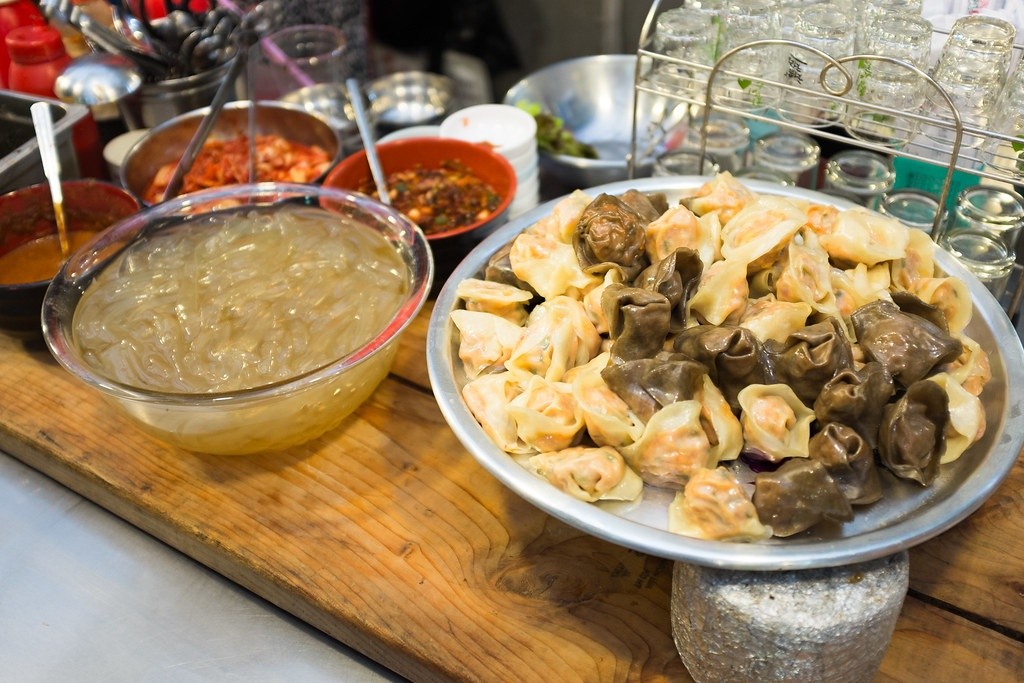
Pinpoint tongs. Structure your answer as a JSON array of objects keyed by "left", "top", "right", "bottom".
[{"left": 161, "top": 28, "right": 275, "bottom": 202}]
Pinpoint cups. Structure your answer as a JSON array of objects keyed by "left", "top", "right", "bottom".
[
  {"left": 652, "top": 115, "right": 1024, "bottom": 304},
  {"left": 248, "top": 25, "right": 348, "bottom": 187},
  {"left": 651, "top": 0, "right": 1024, "bottom": 148},
  {"left": 84, "top": 16, "right": 239, "bottom": 132}
]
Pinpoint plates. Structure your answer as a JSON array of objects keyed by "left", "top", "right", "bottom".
[{"left": 425, "top": 174, "right": 1024, "bottom": 572}]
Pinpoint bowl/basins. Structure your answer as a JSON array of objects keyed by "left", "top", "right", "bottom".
[
  {"left": 120, "top": 100, "right": 343, "bottom": 209},
  {"left": 0, "top": 181, "right": 140, "bottom": 353},
  {"left": 439, "top": 104, "right": 540, "bottom": 236},
  {"left": 318, "top": 138, "right": 517, "bottom": 298},
  {"left": 363, "top": 72, "right": 455, "bottom": 128},
  {"left": 103, "top": 129, "right": 152, "bottom": 183},
  {"left": 503, "top": 54, "right": 706, "bottom": 189},
  {"left": 38, "top": 182, "right": 435, "bottom": 457}
]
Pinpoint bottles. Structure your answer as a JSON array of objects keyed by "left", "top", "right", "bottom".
[{"left": 0, "top": 0, "right": 70, "bottom": 97}]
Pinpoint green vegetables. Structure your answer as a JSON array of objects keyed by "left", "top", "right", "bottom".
[{"left": 516, "top": 98, "right": 599, "bottom": 160}]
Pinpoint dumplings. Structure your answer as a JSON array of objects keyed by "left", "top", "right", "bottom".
[{"left": 451, "top": 175, "right": 991, "bottom": 547}]
